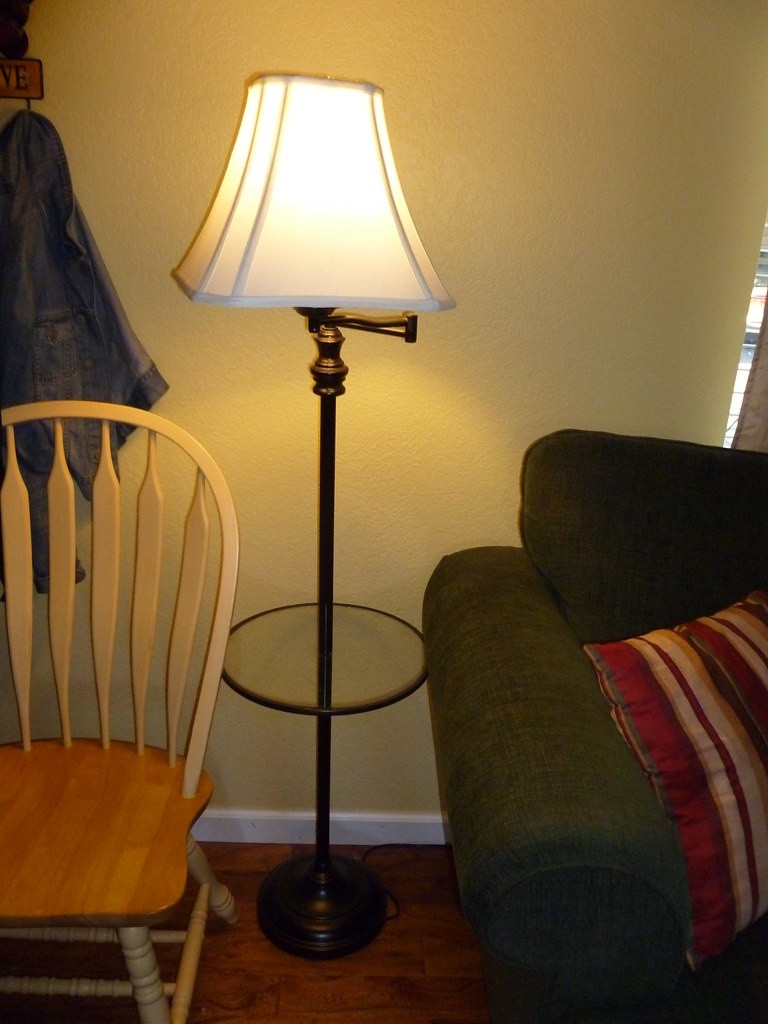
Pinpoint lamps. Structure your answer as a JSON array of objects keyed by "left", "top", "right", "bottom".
[{"left": 169, "top": 72, "right": 457, "bottom": 964}]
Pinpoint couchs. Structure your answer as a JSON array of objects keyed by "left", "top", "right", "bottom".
[{"left": 421, "top": 427, "right": 768, "bottom": 1024}]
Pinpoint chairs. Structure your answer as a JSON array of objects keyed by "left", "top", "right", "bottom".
[{"left": 0, "top": 400, "right": 239, "bottom": 1024}]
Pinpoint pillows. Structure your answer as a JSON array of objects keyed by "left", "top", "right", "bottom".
[{"left": 579, "top": 587, "right": 768, "bottom": 973}]
